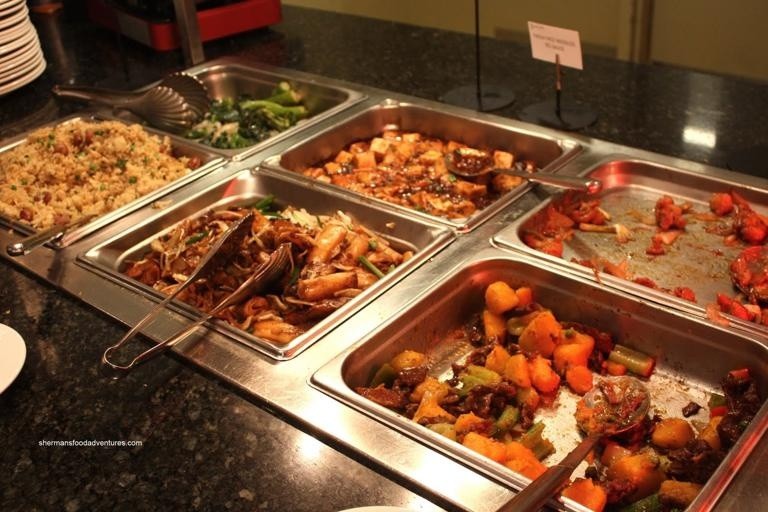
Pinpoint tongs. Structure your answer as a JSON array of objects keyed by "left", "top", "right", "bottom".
[
  {"left": 50, "top": 71, "right": 211, "bottom": 134},
  {"left": 100, "top": 210, "right": 293, "bottom": 380}
]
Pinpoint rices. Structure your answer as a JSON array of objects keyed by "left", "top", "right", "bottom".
[{"left": 0, "top": 121, "right": 201, "bottom": 243}]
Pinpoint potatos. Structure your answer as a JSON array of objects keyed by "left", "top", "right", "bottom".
[{"left": 389, "top": 281, "right": 756, "bottom": 512}]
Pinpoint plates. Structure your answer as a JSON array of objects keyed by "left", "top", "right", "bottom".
[
  {"left": 0, "top": 0, "right": 48, "bottom": 97},
  {"left": 0, "top": 322, "right": 27, "bottom": 399}
]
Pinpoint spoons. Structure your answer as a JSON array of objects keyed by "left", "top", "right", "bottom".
[
  {"left": 445, "top": 147, "right": 602, "bottom": 194},
  {"left": 493, "top": 375, "right": 651, "bottom": 512}
]
